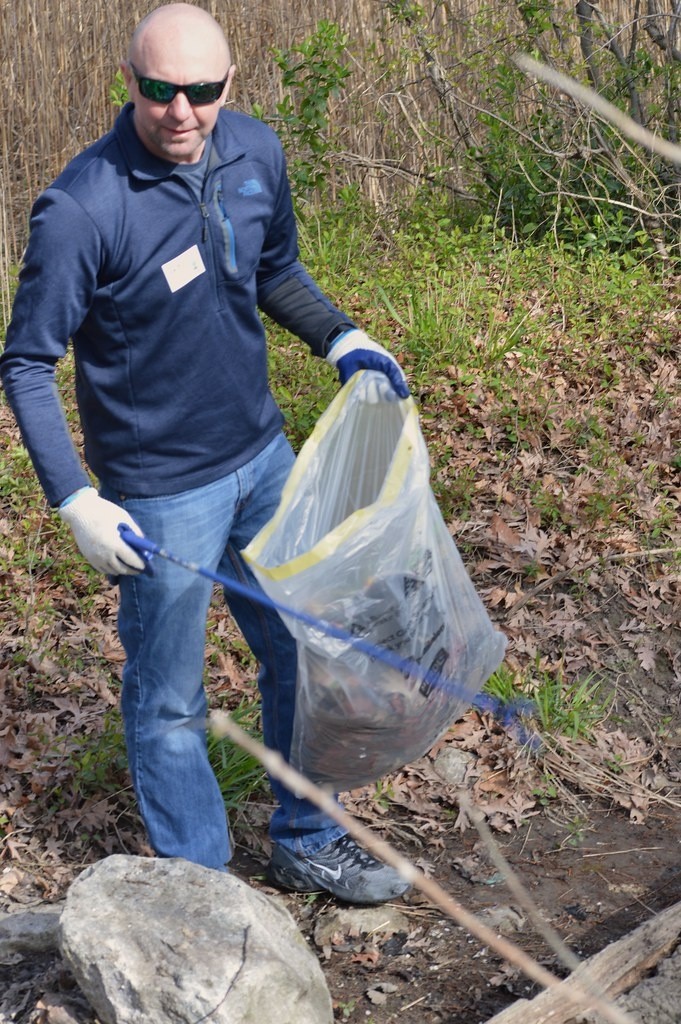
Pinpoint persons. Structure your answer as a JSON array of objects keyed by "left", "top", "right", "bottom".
[{"left": 0, "top": 3, "right": 412, "bottom": 909}]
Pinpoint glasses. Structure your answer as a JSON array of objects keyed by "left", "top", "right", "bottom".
[{"left": 130, "top": 63, "right": 228, "bottom": 105}]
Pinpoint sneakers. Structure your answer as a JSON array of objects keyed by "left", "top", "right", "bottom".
[{"left": 270, "top": 833, "right": 413, "bottom": 903}]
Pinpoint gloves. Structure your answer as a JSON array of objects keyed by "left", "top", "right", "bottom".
[
  {"left": 59, "top": 485, "right": 154, "bottom": 578},
  {"left": 326, "top": 328, "right": 410, "bottom": 403}
]
